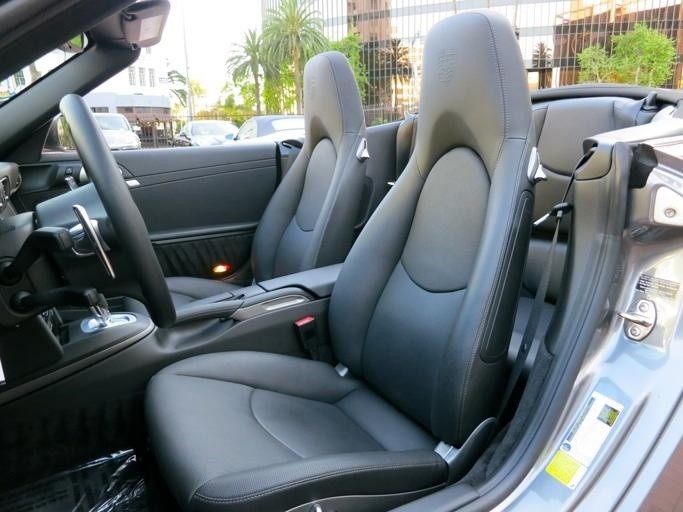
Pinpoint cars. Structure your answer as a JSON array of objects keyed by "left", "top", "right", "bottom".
[
  {"left": 91, "top": 111, "right": 144, "bottom": 151},
  {"left": 1, "top": 0, "right": 682, "bottom": 512},
  {"left": 224, "top": 113, "right": 307, "bottom": 148},
  {"left": 170, "top": 118, "right": 240, "bottom": 149}
]
